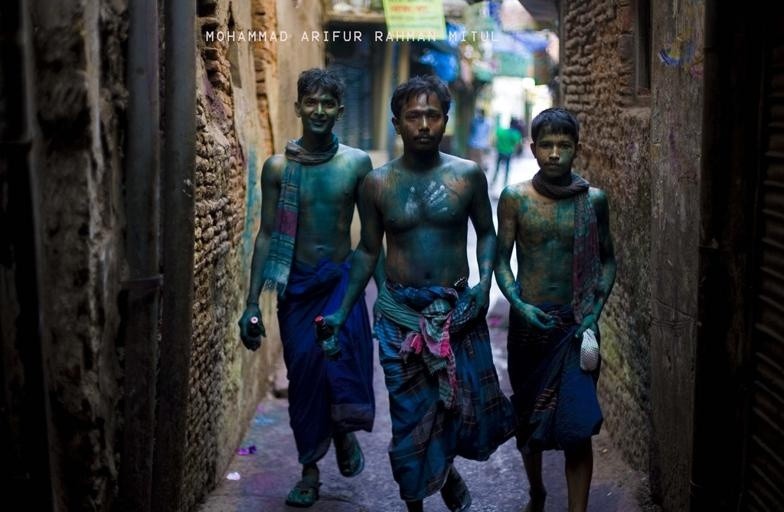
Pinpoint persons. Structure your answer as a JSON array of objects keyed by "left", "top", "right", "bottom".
[
  {"left": 466, "top": 109, "right": 494, "bottom": 171},
  {"left": 239, "top": 66, "right": 386, "bottom": 505},
  {"left": 316, "top": 75, "right": 514, "bottom": 511},
  {"left": 494, "top": 108, "right": 619, "bottom": 512},
  {"left": 492, "top": 118, "right": 524, "bottom": 187}
]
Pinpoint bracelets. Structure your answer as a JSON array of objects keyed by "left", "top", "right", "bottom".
[{"left": 246, "top": 302, "right": 259, "bottom": 307}]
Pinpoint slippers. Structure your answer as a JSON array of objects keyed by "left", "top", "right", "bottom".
[
  {"left": 332, "top": 433, "right": 363, "bottom": 477},
  {"left": 286, "top": 481, "right": 319, "bottom": 507},
  {"left": 439, "top": 468, "right": 471, "bottom": 512}
]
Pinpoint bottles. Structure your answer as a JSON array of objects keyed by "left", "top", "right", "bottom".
[
  {"left": 247, "top": 317, "right": 262, "bottom": 353},
  {"left": 315, "top": 316, "right": 343, "bottom": 362}
]
